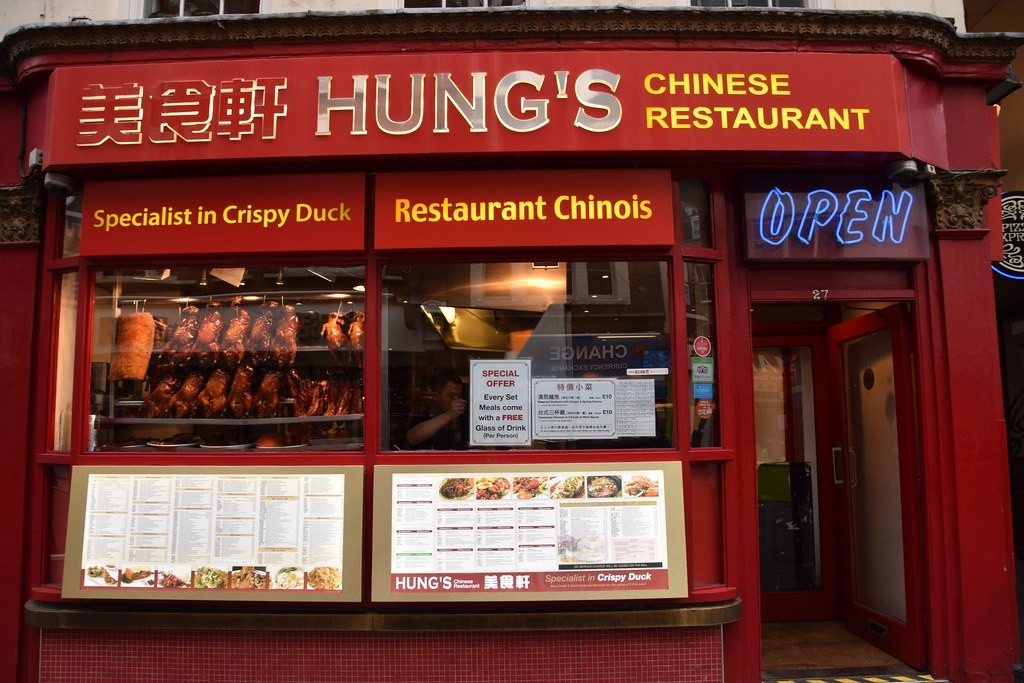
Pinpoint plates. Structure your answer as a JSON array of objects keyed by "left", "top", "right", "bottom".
[
  {"left": 233, "top": 570, "right": 266, "bottom": 575},
  {"left": 256, "top": 444, "right": 306, "bottom": 448},
  {"left": 105, "top": 564, "right": 117, "bottom": 570},
  {"left": 89, "top": 576, "right": 104, "bottom": 585},
  {"left": 103, "top": 445, "right": 145, "bottom": 448},
  {"left": 147, "top": 443, "right": 196, "bottom": 447},
  {"left": 200, "top": 445, "right": 252, "bottom": 449}
]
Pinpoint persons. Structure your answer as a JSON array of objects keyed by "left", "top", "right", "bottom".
[{"left": 406, "top": 372, "right": 467, "bottom": 451}]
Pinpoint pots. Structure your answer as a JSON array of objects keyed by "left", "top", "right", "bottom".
[{"left": 587, "top": 476, "right": 621, "bottom": 498}]
